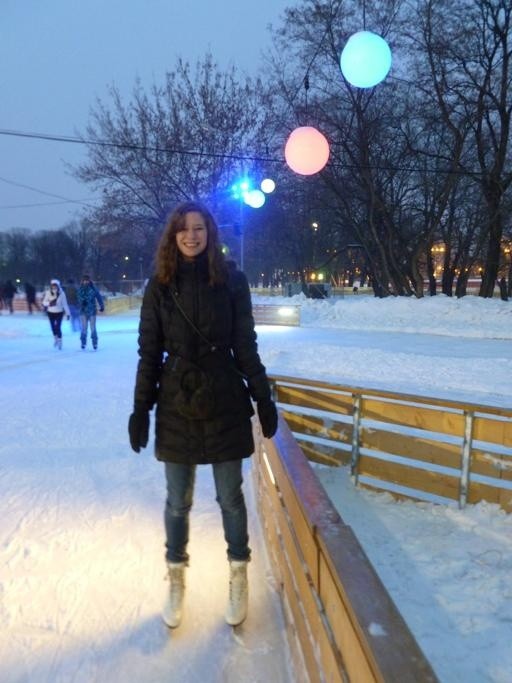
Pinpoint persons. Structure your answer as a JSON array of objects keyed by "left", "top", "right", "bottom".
[
  {"left": 24, "top": 283, "right": 41, "bottom": 315},
  {"left": 497, "top": 265, "right": 508, "bottom": 301},
  {"left": 249, "top": 270, "right": 300, "bottom": 288},
  {"left": 1, "top": 279, "right": 18, "bottom": 312},
  {"left": 352, "top": 280, "right": 360, "bottom": 294},
  {"left": 127, "top": 199, "right": 279, "bottom": 629},
  {"left": 38, "top": 273, "right": 104, "bottom": 350}
]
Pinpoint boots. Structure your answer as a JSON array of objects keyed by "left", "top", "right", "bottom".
[
  {"left": 163, "top": 552, "right": 190, "bottom": 628},
  {"left": 53, "top": 335, "right": 62, "bottom": 347},
  {"left": 225, "top": 555, "right": 250, "bottom": 626}
]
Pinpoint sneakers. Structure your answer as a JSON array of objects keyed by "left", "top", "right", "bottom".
[{"left": 82, "top": 344, "right": 98, "bottom": 349}]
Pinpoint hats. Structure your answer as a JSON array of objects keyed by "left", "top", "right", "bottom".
[{"left": 51, "top": 279, "right": 60, "bottom": 287}]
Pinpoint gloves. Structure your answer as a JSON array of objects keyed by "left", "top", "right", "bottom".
[
  {"left": 129, "top": 413, "right": 149, "bottom": 452},
  {"left": 257, "top": 400, "right": 277, "bottom": 438}
]
